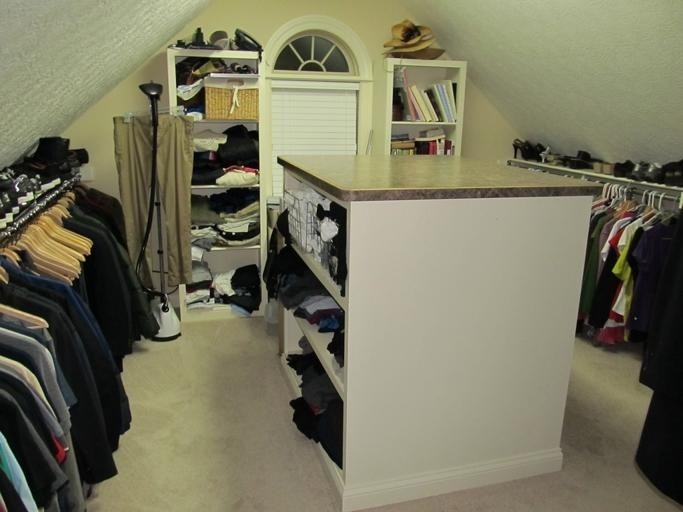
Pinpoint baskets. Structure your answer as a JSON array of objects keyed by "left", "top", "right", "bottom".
[
  {"left": 282, "top": 189, "right": 305, "bottom": 252},
  {"left": 307, "top": 201, "right": 341, "bottom": 284},
  {"left": 204, "top": 77, "right": 259, "bottom": 121}
]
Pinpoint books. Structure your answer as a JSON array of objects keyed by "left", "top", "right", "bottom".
[{"left": 391, "top": 78, "right": 457, "bottom": 155}]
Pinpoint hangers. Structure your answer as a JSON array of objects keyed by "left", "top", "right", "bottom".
[
  {"left": 591, "top": 181, "right": 681, "bottom": 240},
  {"left": 1, "top": 174, "right": 120, "bottom": 410}
]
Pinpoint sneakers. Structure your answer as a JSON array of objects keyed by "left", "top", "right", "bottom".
[
  {"left": 563, "top": 151, "right": 682, "bottom": 187},
  {"left": 0, "top": 135, "right": 89, "bottom": 230}
]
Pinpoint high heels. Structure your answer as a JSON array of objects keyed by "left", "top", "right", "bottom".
[{"left": 513, "top": 138, "right": 560, "bottom": 163}]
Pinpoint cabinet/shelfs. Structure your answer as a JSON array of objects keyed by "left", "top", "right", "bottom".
[
  {"left": 165, "top": 45, "right": 267, "bottom": 323},
  {"left": 372, "top": 56, "right": 467, "bottom": 157},
  {"left": 279, "top": 167, "right": 593, "bottom": 512}
]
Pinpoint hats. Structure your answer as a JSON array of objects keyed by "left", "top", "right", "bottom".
[
  {"left": 233, "top": 28, "right": 261, "bottom": 50},
  {"left": 382, "top": 19, "right": 442, "bottom": 58}
]
